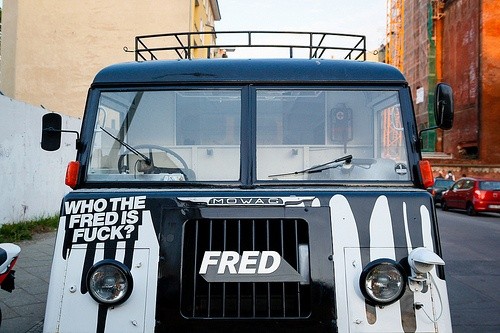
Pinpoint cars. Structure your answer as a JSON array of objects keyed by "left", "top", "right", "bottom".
[
  {"left": 426, "top": 177, "right": 456, "bottom": 205},
  {"left": 442, "top": 176, "right": 500, "bottom": 216}
]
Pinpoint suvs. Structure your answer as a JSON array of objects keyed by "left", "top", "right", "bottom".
[{"left": 40, "top": 29, "right": 455, "bottom": 333}]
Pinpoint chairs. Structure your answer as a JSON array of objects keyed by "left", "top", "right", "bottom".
[
  {"left": 146, "top": 168, "right": 197, "bottom": 182},
  {"left": 336, "top": 158, "right": 400, "bottom": 180}
]
